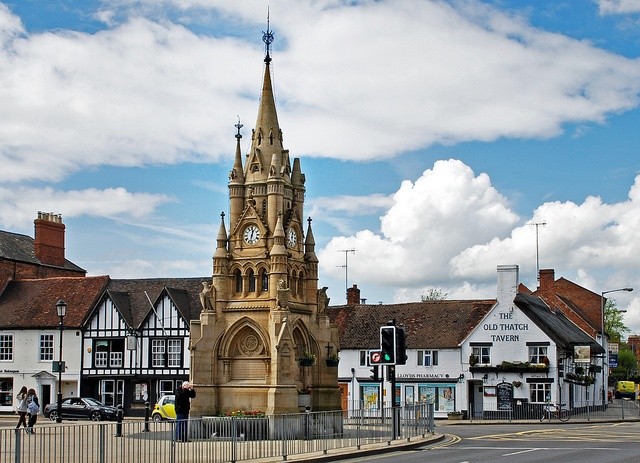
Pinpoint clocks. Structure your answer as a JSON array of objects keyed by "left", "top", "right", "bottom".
[
  {"left": 286, "top": 226, "right": 299, "bottom": 248},
  {"left": 241, "top": 221, "right": 262, "bottom": 245}
]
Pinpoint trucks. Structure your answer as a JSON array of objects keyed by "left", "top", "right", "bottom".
[{"left": 615, "top": 380, "right": 635, "bottom": 400}]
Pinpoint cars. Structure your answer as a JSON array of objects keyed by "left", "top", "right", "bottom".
[
  {"left": 151, "top": 393, "right": 177, "bottom": 422},
  {"left": 44, "top": 396, "right": 124, "bottom": 421}
]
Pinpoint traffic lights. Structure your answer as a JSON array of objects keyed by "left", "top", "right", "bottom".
[
  {"left": 380, "top": 326, "right": 396, "bottom": 364},
  {"left": 395, "top": 325, "right": 408, "bottom": 365},
  {"left": 370, "top": 365, "right": 377, "bottom": 380}
]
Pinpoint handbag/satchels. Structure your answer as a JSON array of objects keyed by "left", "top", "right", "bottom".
[{"left": 17, "top": 397, "right": 27, "bottom": 411}]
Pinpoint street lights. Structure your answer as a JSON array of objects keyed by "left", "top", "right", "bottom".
[
  {"left": 56, "top": 297, "right": 67, "bottom": 422},
  {"left": 600, "top": 287, "right": 633, "bottom": 411}
]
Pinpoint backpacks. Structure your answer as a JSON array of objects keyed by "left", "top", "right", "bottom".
[{"left": 28, "top": 395, "right": 39, "bottom": 418}]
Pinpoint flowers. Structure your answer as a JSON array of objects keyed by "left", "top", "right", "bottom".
[
  {"left": 300, "top": 350, "right": 317, "bottom": 367},
  {"left": 297, "top": 385, "right": 311, "bottom": 395}
]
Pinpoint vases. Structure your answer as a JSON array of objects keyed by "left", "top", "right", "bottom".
[
  {"left": 299, "top": 358, "right": 313, "bottom": 366},
  {"left": 298, "top": 395, "right": 312, "bottom": 407}
]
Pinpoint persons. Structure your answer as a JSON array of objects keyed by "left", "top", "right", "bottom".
[
  {"left": 15, "top": 385, "right": 30, "bottom": 434},
  {"left": 174, "top": 381, "right": 196, "bottom": 442},
  {"left": 25, "top": 388, "right": 40, "bottom": 434}
]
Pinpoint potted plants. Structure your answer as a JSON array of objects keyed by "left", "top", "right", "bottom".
[{"left": 326, "top": 352, "right": 340, "bottom": 367}]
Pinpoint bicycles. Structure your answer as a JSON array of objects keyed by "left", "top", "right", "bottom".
[{"left": 540, "top": 400, "right": 570, "bottom": 421}]
